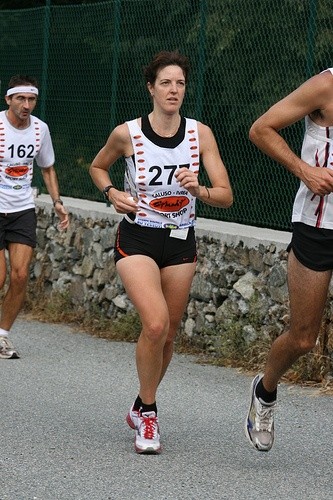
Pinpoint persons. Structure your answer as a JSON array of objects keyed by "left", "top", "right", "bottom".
[
  {"left": 0, "top": 75, "right": 69, "bottom": 358},
  {"left": 90, "top": 50, "right": 233, "bottom": 454},
  {"left": 244, "top": 68, "right": 333, "bottom": 452}
]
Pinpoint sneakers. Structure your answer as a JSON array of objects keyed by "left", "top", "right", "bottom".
[
  {"left": 0, "top": 335, "right": 20, "bottom": 359},
  {"left": 245, "top": 374, "right": 278, "bottom": 453},
  {"left": 134, "top": 408, "right": 162, "bottom": 455},
  {"left": 126, "top": 402, "right": 161, "bottom": 439}
]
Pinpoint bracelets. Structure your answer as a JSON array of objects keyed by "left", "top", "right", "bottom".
[
  {"left": 205, "top": 186, "right": 210, "bottom": 204},
  {"left": 103, "top": 185, "right": 115, "bottom": 201},
  {"left": 53, "top": 199, "right": 63, "bottom": 208}
]
